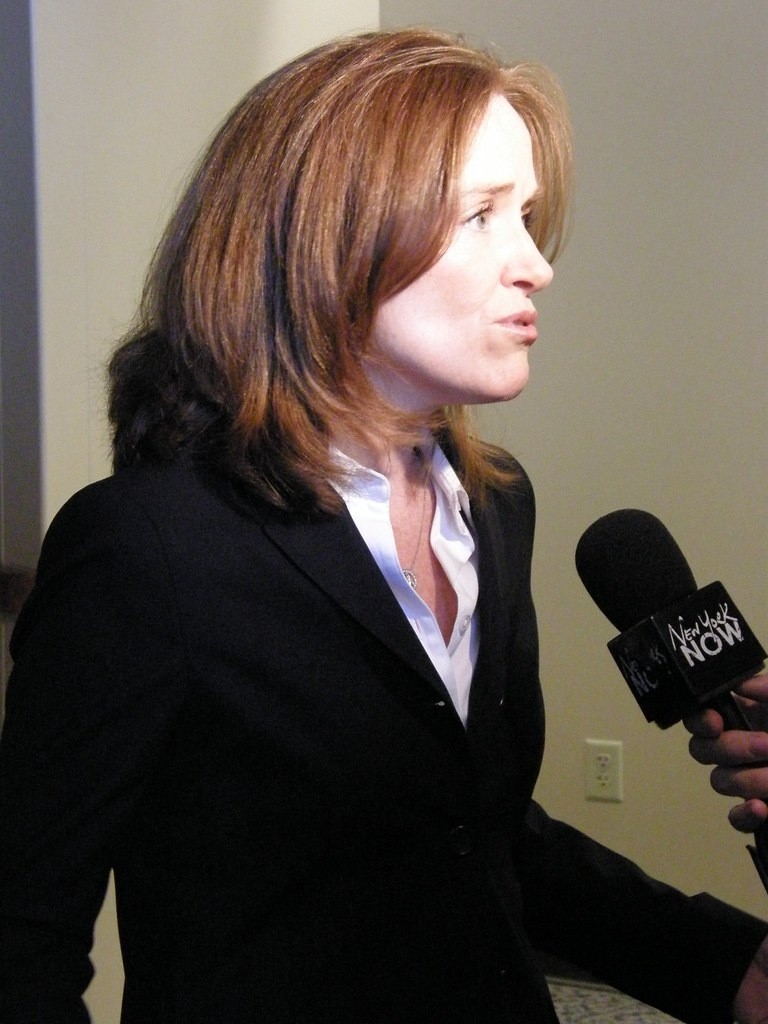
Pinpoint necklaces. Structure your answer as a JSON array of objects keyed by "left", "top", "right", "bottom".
[{"left": 398, "top": 484, "right": 427, "bottom": 589}]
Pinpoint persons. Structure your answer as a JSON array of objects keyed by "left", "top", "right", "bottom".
[{"left": 0, "top": 32, "right": 768, "bottom": 1024}]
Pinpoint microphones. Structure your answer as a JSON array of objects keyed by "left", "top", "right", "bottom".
[{"left": 575, "top": 509, "right": 768, "bottom": 892}]
